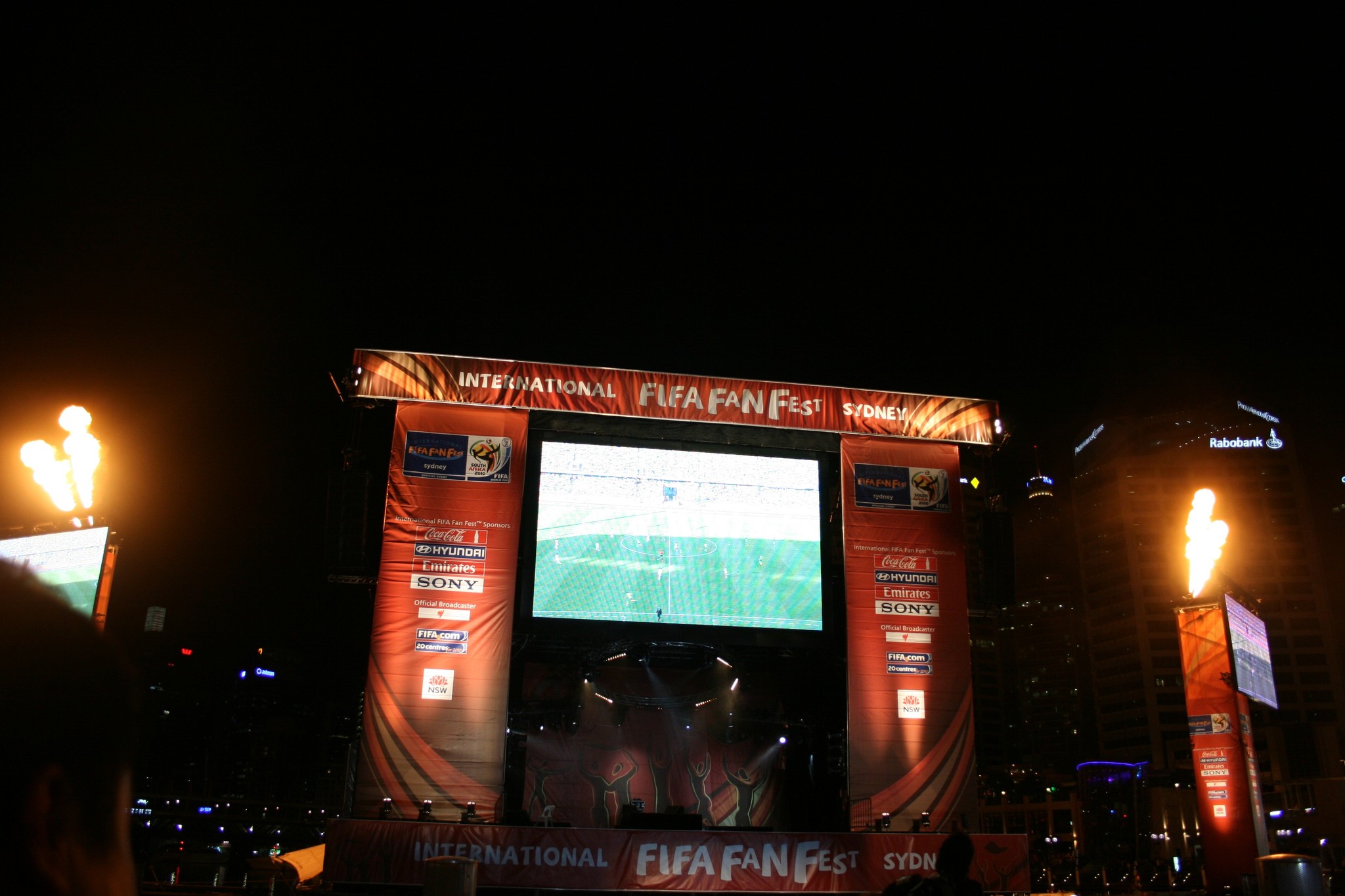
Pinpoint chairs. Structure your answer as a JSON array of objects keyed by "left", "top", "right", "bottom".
[{"left": 535, "top": 805, "right": 556, "bottom": 827}]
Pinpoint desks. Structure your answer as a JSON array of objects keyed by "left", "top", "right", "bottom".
[{"left": 630, "top": 813, "right": 702, "bottom": 830}]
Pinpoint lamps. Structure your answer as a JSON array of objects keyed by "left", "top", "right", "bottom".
[
  {"left": 377, "top": 796, "right": 393, "bottom": 820},
  {"left": 881, "top": 811, "right": 892, "bottom": 832},
  {"left": 417, "top": 798, "right": 432, "bottom": 821},
  {"left": 460, "top": 800, "right": 477, "bottom": 824},
  {"left": 920, "top": 811, "right": 932, "bottom": 834}
]
